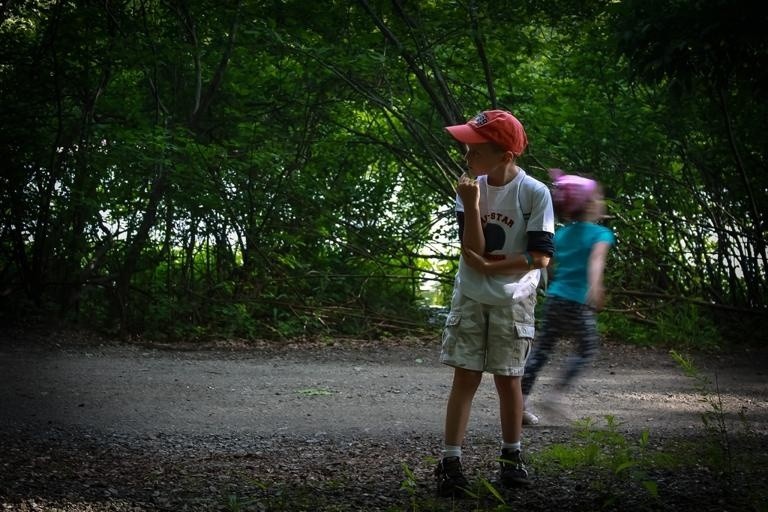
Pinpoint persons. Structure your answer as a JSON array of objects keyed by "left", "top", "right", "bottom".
[
  {"left": 519, "top": 169, "right": 612, "bottom": 426},
  {"left": 438, "top": 109, "right": 556, "bottom": 498}
]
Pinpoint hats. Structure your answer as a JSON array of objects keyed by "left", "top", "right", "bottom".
[
  {"left": 442, "top": 110, "right": 529, "bottom": 158},
  {"left": 545, "top": 175, "right": 598, "bottom": 210}
]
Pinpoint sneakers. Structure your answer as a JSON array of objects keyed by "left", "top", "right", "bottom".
[
  {"left": 499, "top": 448, "right": 529, "bottom": 485},
  {"left": 435, "top": 456, "right": 467, "bottom": 496}
]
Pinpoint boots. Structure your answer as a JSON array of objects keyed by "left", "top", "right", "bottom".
[{"left": 520, "top": 394, "right": 540, "bottom": 425}]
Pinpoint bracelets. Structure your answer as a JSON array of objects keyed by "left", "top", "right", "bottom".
[{"left": 522, "top": 249, "right": 535, "bottom": 273}]
